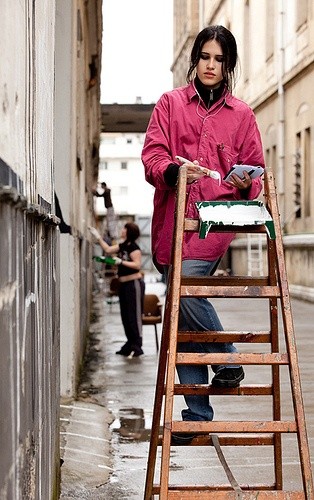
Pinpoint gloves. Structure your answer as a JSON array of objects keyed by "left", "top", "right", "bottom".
[
  {"left": 112, "top": 257, "right": 122, "bottom": 264},
  {"left": 88, "top": 226, "right": 100, "bottom": 243}
]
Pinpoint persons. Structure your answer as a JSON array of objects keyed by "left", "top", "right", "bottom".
[
  {"left": 90, "top": 182, "right": 116, "bottom": 239},
  {"left": 143, "top": 26, "right": 264, "bottom": 445},
  {"left": 86, "top": 223, "right": 145, "bottom": 359}
]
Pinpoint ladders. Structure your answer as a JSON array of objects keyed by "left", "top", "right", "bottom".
[
  {"left": 144, "top": 167, "right": 314, "bottom": 500},
  {"left": 99, "top": 214, "right": 119, "bottom": 299}
]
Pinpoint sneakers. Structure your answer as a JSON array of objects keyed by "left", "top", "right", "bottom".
[
  {"left": 212, "top": 366, "right": 245, "bottom": 387},
  {"left": 171, "top": 433, "right": 209, "bottom": 445}
]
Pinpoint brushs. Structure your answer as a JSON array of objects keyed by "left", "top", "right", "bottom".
[{"left": 175, "top": 156, "right": 220, "bottom": 180}]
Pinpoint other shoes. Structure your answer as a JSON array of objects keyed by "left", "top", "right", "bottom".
[{"left": 133, "top": 349, "right": 144, "bottom": 357}]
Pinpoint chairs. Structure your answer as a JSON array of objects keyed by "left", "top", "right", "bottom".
[
  {"left": 104, "top": 270, "right": 120, "bottom": 313},
  {"left": 142, "top": 295, "right": 162, "bottom": 356}
]
